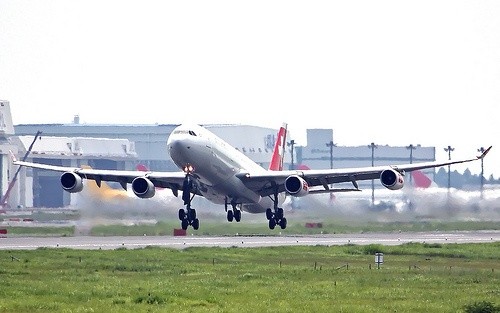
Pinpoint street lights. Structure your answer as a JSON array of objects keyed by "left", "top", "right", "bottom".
[
  {"left": 367, "top": 143, "right": 378, "bottom": 205},
  {"left": 444, "top": 146, "right": 454, "bottom": 188},
  {"left": 477, "top": 147, "right": 485, "bottom": 193},
  {"left": 406, "top": 144, "right": 416, "bottom": 184},
  {"left": 286, "top": 140, "right": 296, "bottom": 170},
  {"left": 326, "top": 141, "right": 337, "bottom": 198}
]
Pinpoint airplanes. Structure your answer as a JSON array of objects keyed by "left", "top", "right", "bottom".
[{"left": 9, "top": 124, "right": 492, "bottom": 230}]
